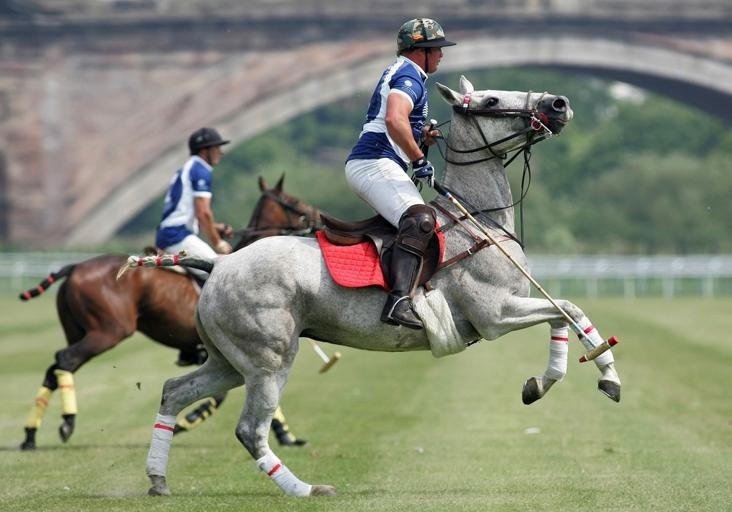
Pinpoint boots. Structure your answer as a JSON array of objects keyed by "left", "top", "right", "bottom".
[{"left": 380, "top": 202, "right": 437, "bottom": 330}]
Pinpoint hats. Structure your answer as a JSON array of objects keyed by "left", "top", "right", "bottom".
[
  {"left": 397, "top": 17, "right": 457, "bottom": 50},
  {"left": 188, "top": 127, "right": 231, "bottom": 152}
]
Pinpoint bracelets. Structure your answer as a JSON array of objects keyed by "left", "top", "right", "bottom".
[{"left": 412, "top": 158, "right": 427, "bottom": 168}]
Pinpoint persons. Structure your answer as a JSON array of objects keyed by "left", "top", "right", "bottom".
[
  {"left": 342, "top": 17, "right": 458, "bottom": 329},
  {"left": 156, "top": 127, "right": 236, "bottom": 366}
]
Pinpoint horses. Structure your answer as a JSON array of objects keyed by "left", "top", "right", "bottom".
[
  {"left": 18, "top": 172, "right": 333, "bottom": 452},
  {"left": 143, "top": 74, "right": 622, "bottom": 499}
]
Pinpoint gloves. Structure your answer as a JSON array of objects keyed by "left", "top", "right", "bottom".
[
  {"left": 213, "top": 239, "right": 232, "bottom": 254},
  {"left": 411, "top": 155, "right": 434, "bottom": 179}
]
